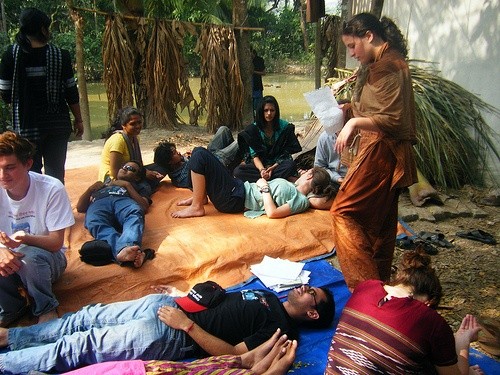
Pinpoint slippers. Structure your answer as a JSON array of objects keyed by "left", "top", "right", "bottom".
[
  {"left": 419, "top": 230, "right": 454, "bottom": 248},
  {"left": 457, "top": 228, "right": 496, "bottom": 244}
]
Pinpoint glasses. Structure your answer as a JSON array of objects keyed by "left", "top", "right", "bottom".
[
  {"left": 307, "top": 285, "right": 319, "bottom": 313},
  {"left": 123, "top": 165, "right": 135, "bottom": 173}
]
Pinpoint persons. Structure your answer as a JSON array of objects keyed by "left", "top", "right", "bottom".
[
  {"left": 170, "top": 145, "right": 340, "bottom": 221},
  {"left": 0, "top": 6, "right": 84, "bottom": 185},
  {"left": 152, "top": 125, "right": 241, "bottom": 192},
  {"left": 0, "top": 282, "right": 336, "bottom": 375},
  {"left": 56, "top": 328, "right": 299, "bottom": 375},
  {"left": 0, "top": 129, "right": 78, "bottom": 330},
  {"left": 76, "top": 160, "right": 152, "bottom": 270},
  {"left": 330, "top": 10, "right": 421, "bottom": 294},
  {"left": 97, "top": 105, "right": 169, "bottom": 206},
  {"left": 232, "top": 95, "right": 302, "bottom": 184},
  {"left": 323, "top": 245, "right": 487, "bottom": 375},
  {"left": 305, "top": 99, "right": 361, "bottom": 210}
]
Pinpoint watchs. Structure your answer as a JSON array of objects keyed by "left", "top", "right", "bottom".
[{"left": 260, "top": 187, "right": 270, "bottom": 193}]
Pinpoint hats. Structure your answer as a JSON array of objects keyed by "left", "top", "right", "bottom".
[{"left": 175, "top": 281, "right": 226, "bottom": 314}]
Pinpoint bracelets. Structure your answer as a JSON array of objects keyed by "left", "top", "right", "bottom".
[
  {"left": 260, "top": 168, "right": 267, "bottom": 173},
  {"left": 261, "top": 185, "right": 269, "bottom": 188},
  {"left": 186, "top": 321, "right": 195, "bottom": 334}
]
